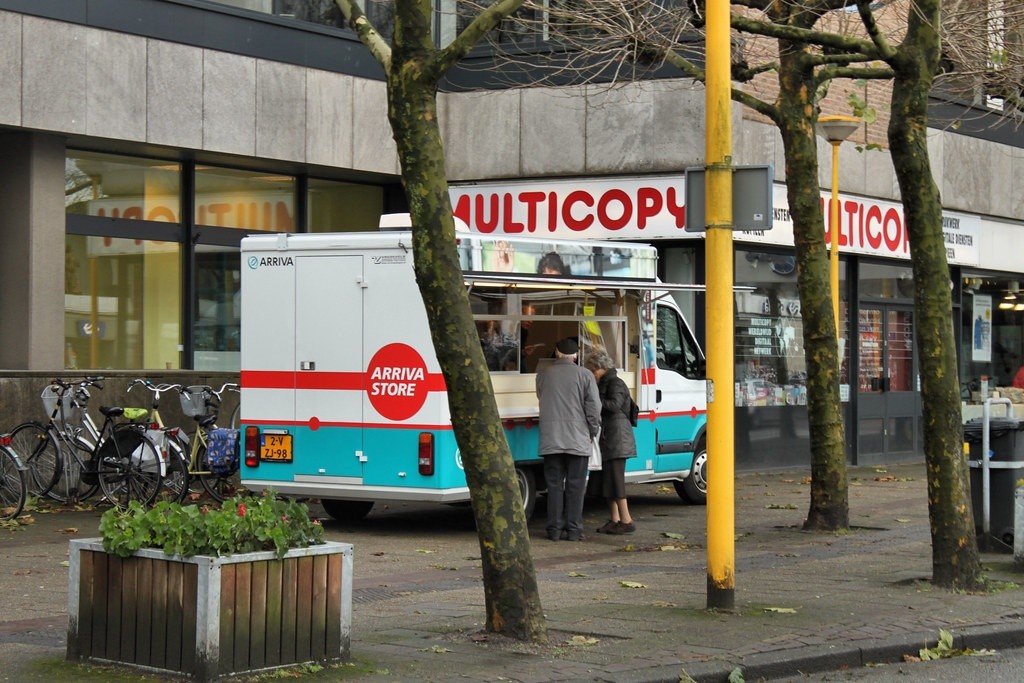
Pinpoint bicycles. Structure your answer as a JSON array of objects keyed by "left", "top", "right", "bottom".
[{"left": 0, "top": 376, "right": 241, "bottom": 521}]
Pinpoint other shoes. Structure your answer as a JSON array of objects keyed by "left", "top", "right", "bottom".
[
  {"left": 567, "top": 530, "right": 584, "bottom": 541},
  {"left": 597, "top": 520, "right": 618, "bottom": 533},
  {"left": 606, "top": 521, "right": 635, "bottom": 535},
  {"left": 547, "top": 529, "right": 563, "bottom": 541}
]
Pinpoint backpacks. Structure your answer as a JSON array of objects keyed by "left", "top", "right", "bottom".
[{"left": 605, "top": 375, "right": 639, "bottom": 428}]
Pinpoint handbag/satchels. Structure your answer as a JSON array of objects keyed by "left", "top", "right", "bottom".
[{"left": 209, "top": 425, "right": 239, "bottom": 474}]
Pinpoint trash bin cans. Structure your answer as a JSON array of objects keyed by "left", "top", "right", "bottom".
[{"left": 963, "top": 415, "right": 1024, "bottom": 553}]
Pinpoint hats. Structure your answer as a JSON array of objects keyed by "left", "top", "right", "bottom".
[{"left": 555, "top": 339, "right": 579, "bottom": 355}]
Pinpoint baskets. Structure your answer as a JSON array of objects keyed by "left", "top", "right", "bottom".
[
  {"left": 41, "top": 385, "right": 73, "bottom": 420},
  {"left": 179, "top": 385, "right": 211, "bottom": 418}
]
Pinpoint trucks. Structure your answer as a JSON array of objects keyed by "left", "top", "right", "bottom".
[{"left": 239, "top": 230, "right": 757, "bottom": 522}]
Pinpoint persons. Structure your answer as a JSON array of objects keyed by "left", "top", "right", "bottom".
[
  {"left": 492, "top": 240, "right": 566, "bottom": 274},
  {"left": 480, "top": 319, "right": 503, "bottom": 371},
  {"left": 583, "top": 350, "right": 637, "bottom": 534},
  {"left": 535, "top": 337, "right": 602, "bottom": 541},
  {"left": 520, "top": 300, "right": 545, "bottom": 373},
  {"left": 501, "top": 348, "right": 518, "bottom": 371}
]
